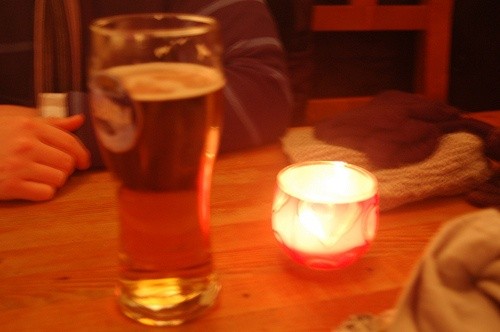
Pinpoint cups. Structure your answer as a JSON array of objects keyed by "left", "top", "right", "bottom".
[{"left": 88, "top": 13, "right": 227, "bottom": 327}]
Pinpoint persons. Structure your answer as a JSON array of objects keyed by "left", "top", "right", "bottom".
[{"left": 0, "top": 0, "right": 296, "bottom": 201}]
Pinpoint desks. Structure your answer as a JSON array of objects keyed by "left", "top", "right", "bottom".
[{"left": 0, "top": 112, "right": 500, "bottom": 332}]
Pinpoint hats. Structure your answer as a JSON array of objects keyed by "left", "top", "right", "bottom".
[{"left": 282, "top": 121, "right": 492, "bottom": 213}]
